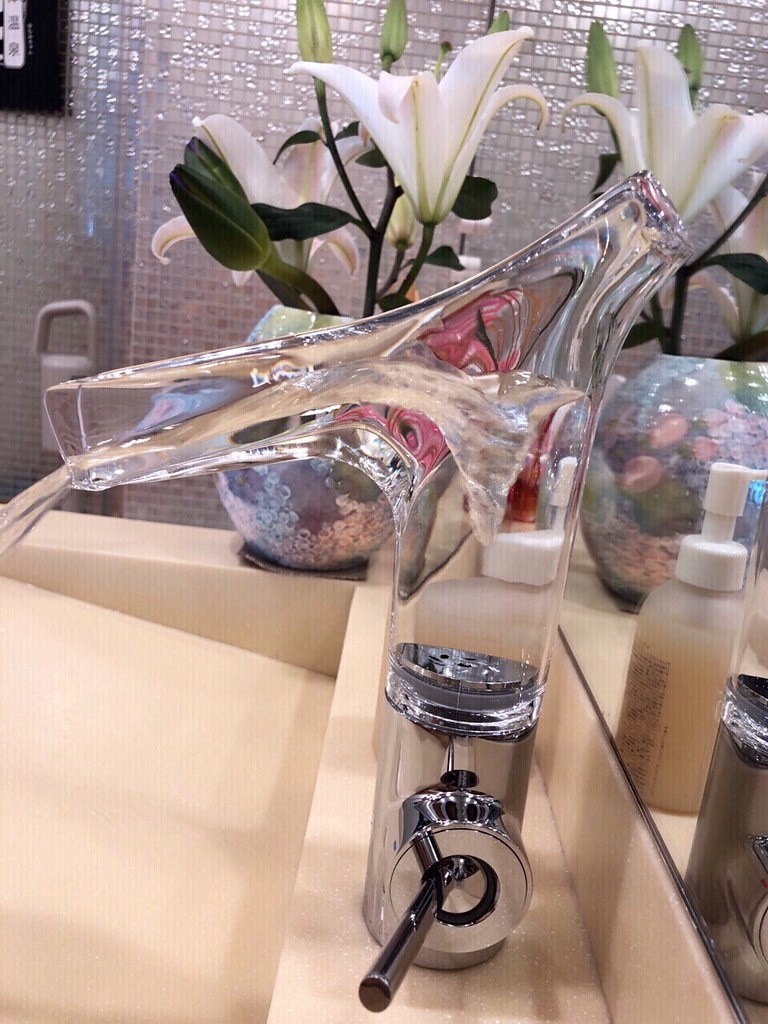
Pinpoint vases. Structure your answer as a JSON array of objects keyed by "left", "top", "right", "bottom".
[
  {"left": 212, "top": 305, "right": 396, "bottom": 581},
  {"left": 578, "top": 356, "right": 768, "bottom": 617}
]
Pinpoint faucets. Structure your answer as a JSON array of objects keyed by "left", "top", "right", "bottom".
[{"left": 36, "top": 171, "right": 689, "bottom": 1016}]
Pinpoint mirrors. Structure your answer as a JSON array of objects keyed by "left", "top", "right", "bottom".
[{"left": 486, "top": 0, "right": 768, "bottom": 1024}]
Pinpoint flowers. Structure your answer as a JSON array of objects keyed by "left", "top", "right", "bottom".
[
  {"left": 561, "top": 21, "right": 768, "bottom": 364},
  {"left": 148, "top": 0, "right": 548, "bottom": 317}
]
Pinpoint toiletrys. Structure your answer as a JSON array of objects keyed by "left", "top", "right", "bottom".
[{"left": 612, "top": 457, "right": 765, "bottom": 812}]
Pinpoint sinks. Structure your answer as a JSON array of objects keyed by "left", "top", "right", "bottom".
[{"left": 0, "top": 548, "right": 356, "bottom": 1024}]
